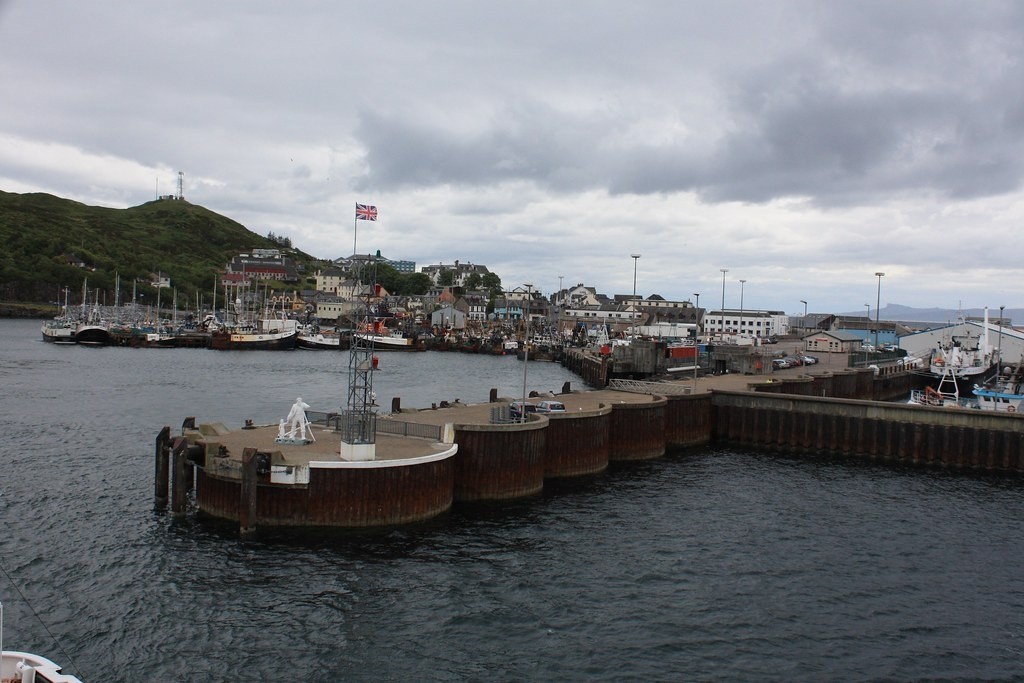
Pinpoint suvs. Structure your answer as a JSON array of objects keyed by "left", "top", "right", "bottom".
[
  {"left": 536, "top": 399, "right": 566, "bottom": 414},
  {"left": 510, "top": 401, "right": 536, "bottom": 423}
]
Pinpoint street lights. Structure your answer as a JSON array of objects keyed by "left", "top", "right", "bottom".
[
  {"left": 801, "top": 300, "right": 807, "bottom": 379},
  {"left": 719, "top": 268, "right": 729, "bottom": 345},
  {"left": 865, "top": 304, "right": 870, "bottom": 369},
  {"left": 524, "top": 284, "right": 533, "bottom": 346},
  {"left": 631, "top": 253, "right": 641, "bottom": 344},
  {"left": 738, "top": 279, "right": 746, "bottom": 335},
  {"left": 693, "top": 292, "right": 700, "bottom": 392},
  {"left": 557, "top": 276, "right": 565, "bottom": 343}
]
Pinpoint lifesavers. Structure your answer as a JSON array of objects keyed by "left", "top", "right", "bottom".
[
  {"left": 1008, "top": 405, "right": 1015, "bottom": 412},
  {"left": 378, "top": 327, "right": 383, "bottom": 333},
  {"left": 933, "top": 359, "right": 944, "bottom": 367},
  {"left": 279, "top": 296, "right": 283, "bottom": 302},
  {"left": 286, "top": 297, "right": 291, "bottom": 303},
  {"left": 272, "top": 296, "right": 277, "bottom": 302}
]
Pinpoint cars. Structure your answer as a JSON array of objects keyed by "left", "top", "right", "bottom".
[
  {"left": 772, "top": 349, "right": 819, "bottom": 370},
  {"left": 861, "top": 343, "right": 898, "bottom": 354}
]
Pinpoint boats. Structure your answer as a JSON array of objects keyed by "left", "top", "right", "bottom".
[
  {"left": 905, "top": 306, "right": 1024, "bottom": 415},
  {"left": 0, "top": 601, "right": 84, "bottom": 683},
  {"left": 39, "top": 271, "right": 410, "bottom": 354}
]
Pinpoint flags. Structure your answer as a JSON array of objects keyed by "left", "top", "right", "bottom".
[{"left": 356, "top": 204, "right": 378, "bottom": 221}]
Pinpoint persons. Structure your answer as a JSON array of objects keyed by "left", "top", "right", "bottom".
[{"left": 279, "top": 397, "right": 310, "bottom": 440}]
